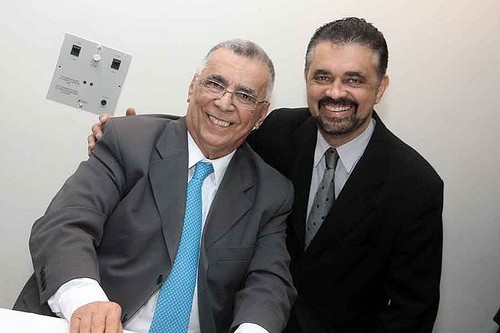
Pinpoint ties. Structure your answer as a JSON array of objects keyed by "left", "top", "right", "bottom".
[
  {"left": 148, "top": 161, "right": 213, "bottom": 333},
  {"left": 302, "top": 148, "right": 339, "bottom": 252}
]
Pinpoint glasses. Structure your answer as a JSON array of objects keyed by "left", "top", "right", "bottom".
[{"left": 197, "top": 76, "right": 266, "bottom": 112}]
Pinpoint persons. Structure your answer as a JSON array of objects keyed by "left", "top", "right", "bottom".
[
  {"left": 11, "top": 38, "right": 294, "bottom": 333},
  {"left": 87, "top": 19, "right": 445, "bottom": 333}
]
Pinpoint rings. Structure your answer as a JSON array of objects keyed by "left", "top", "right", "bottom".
[{"left": 72, "top": 315, "right": 82, "bottom": 319}]
